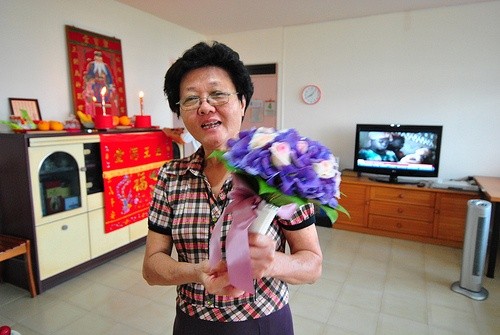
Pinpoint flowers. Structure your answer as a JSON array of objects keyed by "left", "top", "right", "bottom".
[{"left": 205, "top": 126, "right": 351, "bottom": 296}]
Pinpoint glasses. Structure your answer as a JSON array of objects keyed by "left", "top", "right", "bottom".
[{"left": 176, "top": 90, "right": 240, "bottom": 112}]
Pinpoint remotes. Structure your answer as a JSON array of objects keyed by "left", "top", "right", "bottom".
[{"left": 417, "top": 183, "right": 425, "bottom": 187}]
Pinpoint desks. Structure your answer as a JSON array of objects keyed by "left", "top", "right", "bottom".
[{"left": 474, "top": 176, "right": 500, "bottom": 279}]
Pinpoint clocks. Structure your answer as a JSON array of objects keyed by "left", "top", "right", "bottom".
[{"left": 302, "top": 85, "right": 321, "bottom": 105}]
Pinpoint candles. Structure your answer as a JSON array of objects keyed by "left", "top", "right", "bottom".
[
  {"left": 100, "top": 86, "right": 107, "bottom": 116},
  {"left": 139, "top": 91, "right": 144, "bottom": 116}
]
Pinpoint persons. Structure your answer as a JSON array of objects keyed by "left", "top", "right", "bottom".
[
  {"left": 142, "top": 41, "right": 322, "bottom": 335},
  {"left": 359, "top": 131, "right": 430, "bottom": 164}
]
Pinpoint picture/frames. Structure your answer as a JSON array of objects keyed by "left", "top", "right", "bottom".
[{"left": 9, "top": 98, "right": 42, "bottom": 122}]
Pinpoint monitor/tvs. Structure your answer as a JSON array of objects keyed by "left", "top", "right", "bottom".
[{"left": 354, "top": 124, "right": 444, "bottom": 184}]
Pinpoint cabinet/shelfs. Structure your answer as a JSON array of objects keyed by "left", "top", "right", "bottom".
[
  {"left": 0, "top": 127, "right": 186, "bottom": 296},
  {"left": 332, "top": 173, "right": 479, "bottom": 249}
]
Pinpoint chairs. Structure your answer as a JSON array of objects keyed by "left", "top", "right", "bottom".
[{"left": 0, "top": 233, "right": 36, "bottom": 298}]
